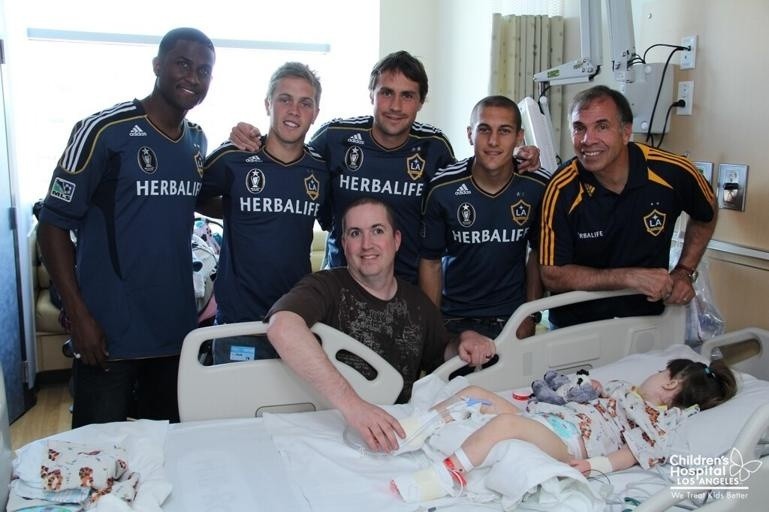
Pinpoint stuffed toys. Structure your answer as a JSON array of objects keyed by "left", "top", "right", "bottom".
[{"left": 529, "top": 370, "right": 602, "bottom": 406}]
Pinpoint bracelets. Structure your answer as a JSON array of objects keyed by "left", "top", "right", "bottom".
[{"left": 673, "top": 262, "right": 699, "bottom": 281}]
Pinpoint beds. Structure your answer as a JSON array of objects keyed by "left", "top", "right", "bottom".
[{"left": 0, "top": 287, "right": 769, "bottom": 512}]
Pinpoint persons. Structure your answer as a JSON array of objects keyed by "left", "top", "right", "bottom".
[
  {"left": 36, "top": 25, "right": 222, "bottom": 431},
  {"left": 415, "top": 93, "right": 558, "bottom": 358},
  {"left": 538, "top": 84, "right": 723, "bottom": 325},
  {"left": 261, "top": 195, "right": 499, "bottom": 456},
  {"left": 364, "top": 357, "right": 740, "bottom": 504},
  {"left": 193, "top": 61, "right": 334, "bottom": 360},
  {"left": 227, "top": 49, "right": 542, "bottom": 288}
]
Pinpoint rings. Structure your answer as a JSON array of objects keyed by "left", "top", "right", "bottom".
[
  {"left": 682, "top": 297, "right": 689, "bottom": 303},
  {"left": 485, "top": 354, "right": 493, "bottom": 360}
]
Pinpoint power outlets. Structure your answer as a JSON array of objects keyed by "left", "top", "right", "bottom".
[{"left": 676, "top": 80, "right": 695, "bottom": 115}]
